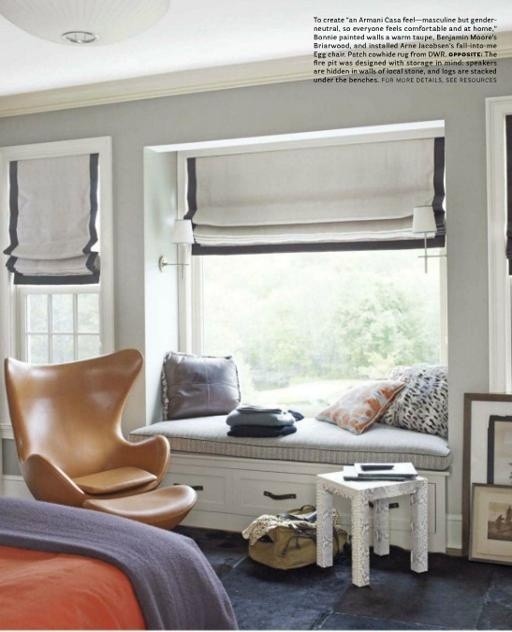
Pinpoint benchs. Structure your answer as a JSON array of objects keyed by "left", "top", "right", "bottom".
[{"left": 128, "top": 414, "right": 450, "bottom": 557}]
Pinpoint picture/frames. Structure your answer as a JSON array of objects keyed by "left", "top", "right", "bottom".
[{"left": 461, "top": 391, "right": 511, "bottom": 569}]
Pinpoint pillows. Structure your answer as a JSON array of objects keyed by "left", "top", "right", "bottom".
[
  {"left": 161, "top": 352, "right": 242, "bottom": 422},
  {"left": 314, "top": 378, "right": 406, "bottom": 436},
  {"left": 373, "top": 363, "right": 449, "bottom": 440}
]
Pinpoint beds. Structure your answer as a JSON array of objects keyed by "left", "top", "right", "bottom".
[{"left": 0, "top": 497, "right": 237, "bottom": 629}]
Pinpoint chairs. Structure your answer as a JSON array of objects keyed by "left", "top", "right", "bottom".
[{"left": 6, "top": 348, "right": 196, "bottom": 531}]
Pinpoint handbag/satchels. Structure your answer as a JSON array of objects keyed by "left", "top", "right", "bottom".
[{"left": 242, "top": 505, "right": 349, "bottom": 571}]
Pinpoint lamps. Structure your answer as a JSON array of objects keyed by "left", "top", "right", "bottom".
[
  {"left": 0, "top": 0, "right": 169, "bottom": 48},
  {"left": 159, "top": 219, "right": 194, "bottom": 273},
  {"left": 412, "top": 204, "right": 447, "bottom": 275}
]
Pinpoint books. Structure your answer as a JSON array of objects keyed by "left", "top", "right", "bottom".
[{"left": 343, "top": 462, "right": 418, "bottom": 481}]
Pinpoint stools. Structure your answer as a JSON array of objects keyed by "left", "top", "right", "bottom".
[{"left": 315, "top": 471, "right": 428, "bottom": 589}]
